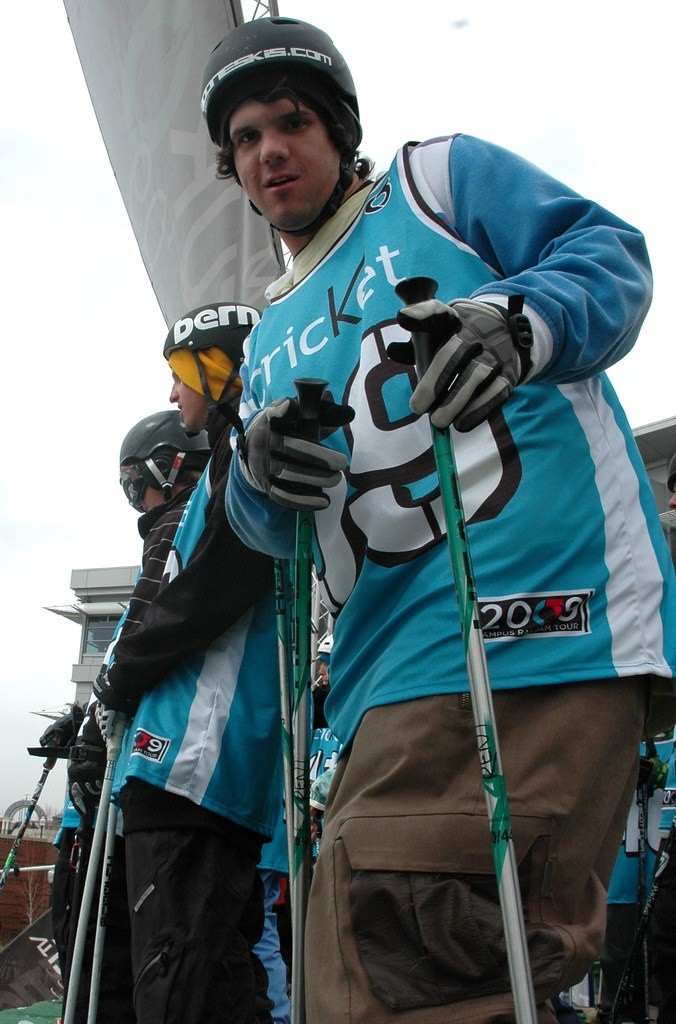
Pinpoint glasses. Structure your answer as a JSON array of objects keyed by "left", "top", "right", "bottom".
[{"left": 120, "top": 464, "right": 148, "bottom": 513}]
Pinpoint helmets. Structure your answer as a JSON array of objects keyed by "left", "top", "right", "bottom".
[
  {"left": 315, "top": 634, "right": 335, "bottom": 653},
  {"left": 201, "top": 18, "right": 363, "bottom": 148},
  {"left": 119, "top": 410, "right": 211, "bottom": 489},
  {"left": 163, "top": 302, "right": 261, "bottom": 360},
  {"left": 667, "top": 451, "right": 676, "bottom": 492}
]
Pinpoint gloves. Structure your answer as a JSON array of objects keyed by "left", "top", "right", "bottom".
[
  {"left": 67, "top": 737, "right": 106, "bottom": 817},
  {"left": 387, "top": 298, "right": 522, "bottom": 432},
  {"left": 242, "top": 390, "right": 355, "bottom": 511},
  {"left": 40, "top": 704, "right": 87, "bottom": 747}
]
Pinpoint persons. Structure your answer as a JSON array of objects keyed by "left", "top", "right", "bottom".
[
  {"left": 196, "top": 17, "right": 676, "bottom": 1024},
  {"left": 40, "top": 303, "right": 662, "bottom": 1024}
]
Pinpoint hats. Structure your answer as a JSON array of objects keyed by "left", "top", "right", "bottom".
[{"left": 168, "top": 347, "right": 242, "bottom": 402}]
